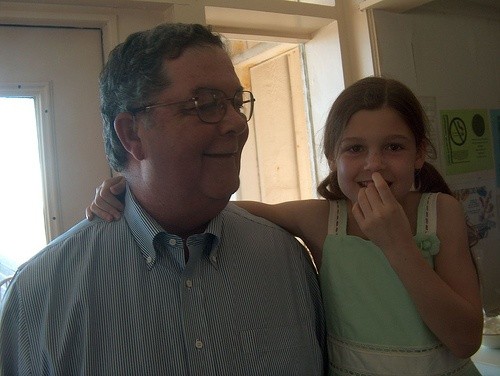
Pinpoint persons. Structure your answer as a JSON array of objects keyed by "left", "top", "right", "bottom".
[
  {"left": 0, "top": 22, "right": 327, "bottom": 376},
  {"left": 85, "top": 76, "right": 484, "bottom": 376}
]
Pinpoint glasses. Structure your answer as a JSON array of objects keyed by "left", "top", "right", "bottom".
[{"left": 127, "top": 90, "right": 256, "bottom": 124}]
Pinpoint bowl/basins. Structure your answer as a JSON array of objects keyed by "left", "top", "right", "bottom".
[{"left": 482, "top": 317, "right": 500, "bottom": 347}]
